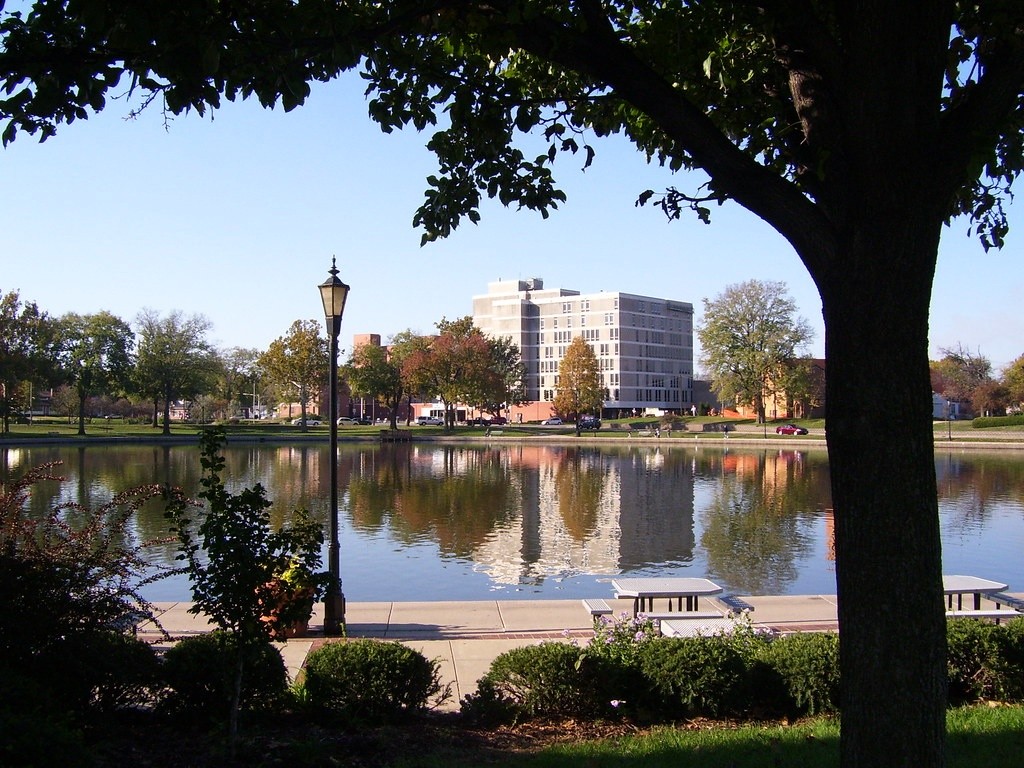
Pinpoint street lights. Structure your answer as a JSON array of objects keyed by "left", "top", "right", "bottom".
[{"left": 314, "top": 254, "right": 348, "bottom": 635}]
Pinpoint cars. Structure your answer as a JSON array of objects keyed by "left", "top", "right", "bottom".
[
  {"left": 337, "top": 417, "right": 359, "bottom": 425},
  {"left": 489, "top": 417, "right": 508, "bottom": 425},
  {"left": 352, "top": 418, "right": 373, "bottom": 426},
  {"left": 776, "top": 424, "right": 809, "bottom": 436},
  {"left": 541, "top": 417, "right": 562, "bottom": 425},
  {"left": 292, "top": 417, "right": 322, "bottom": 426}
]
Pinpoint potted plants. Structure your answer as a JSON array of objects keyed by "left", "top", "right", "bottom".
[{"left": 259, "top": 556, "right": 317, "bottom": 637}]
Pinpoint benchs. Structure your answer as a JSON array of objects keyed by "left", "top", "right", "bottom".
[
  {"left": 636, "top": 611, "right": 722, "bottom": 626},
  {"left": 614, "top": 593, "right": 698, "bottom": 618},
  {"left": 638, "top": 432, "right": 651, "bottom": 437},
  {"left": 981, "top": 591, "right": 1024, "bottom": 611},
  {"left": 49, "top": 432, "right": 58, "bottom": 437},
  {"left": 489, "top": 431, "right": 503, "bottom": 436},
  {"left": 716, "top": 595, "right": 754, "bottom": 614},
  {"left": 583, "top": 599, "right": 613, "bottom": 624}
]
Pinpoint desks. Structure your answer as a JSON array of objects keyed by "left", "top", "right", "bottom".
[
  {"left": 610, "top": 577, "right": 724, "bottom": 620},
  {"left": 942, "top": 575, "right": 1009, "bottom": 621}
]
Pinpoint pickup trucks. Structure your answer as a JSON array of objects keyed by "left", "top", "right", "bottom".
[{"left": 466, "top": 417, "right": 491, "bottom": 427}]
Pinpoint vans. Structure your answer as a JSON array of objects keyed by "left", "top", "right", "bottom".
[{"left": 417, "top": 415, "right": 444, "bottom": 427}]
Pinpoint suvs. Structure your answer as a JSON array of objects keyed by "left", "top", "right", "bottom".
[{"left": 580, "top": 418, "right": 602, "bottom": 429}]
